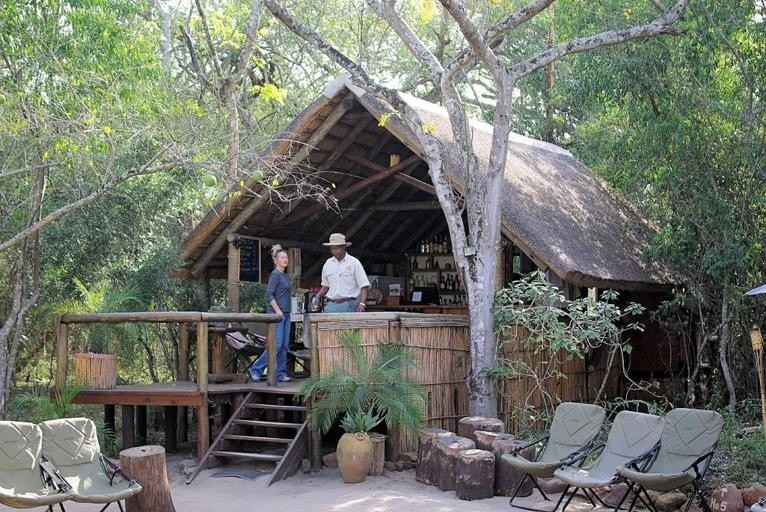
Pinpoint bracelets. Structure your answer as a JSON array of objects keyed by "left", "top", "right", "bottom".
[
  {"left": 315, "top": 295, "right": 322, "bottom": 300},
  {"left": 359, "top": 302, "right": 366, "bottom": 307}
]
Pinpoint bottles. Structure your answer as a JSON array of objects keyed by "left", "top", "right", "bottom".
[
  {"left": 410, "top": 256, "right": 460, "bottom": 289},
  {"left": 417, "top": 235, "right": 448, "bottom": 253},
  {"left": 439, "top": 294, "right": 467, "bottom": 306}
]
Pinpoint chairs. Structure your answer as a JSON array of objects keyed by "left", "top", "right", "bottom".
[
  {"left": 240, "top": 316, "right": 312, "bottom": 378},
  {"left": 611, "top": 408, "right": 725, "bottom": 512},
  {"left": 551, "top": 409, "right": 666, "bottom": 512},
  {"left": 37, "top": 417, "right": 144, "bottom": 512},
  {"left": 499, "top": 401, "right": 606, "bottom": 512},
  {"left": 208, "top": 305, "right": 266, "bottom": 380},
  {"left": 0, "top": 420, "right": 73, "bottom": 512}
]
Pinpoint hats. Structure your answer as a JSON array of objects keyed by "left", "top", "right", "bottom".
[{"left": 322, "top": 233, "right": 353, "bottom": 247}]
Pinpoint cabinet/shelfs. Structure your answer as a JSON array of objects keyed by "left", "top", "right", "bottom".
[{"left": 404, "top": 252, "right": 467, "bottom": 307}]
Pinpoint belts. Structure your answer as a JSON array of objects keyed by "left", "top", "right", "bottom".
[{"left": 327, "top": 298, "right": 356, "bottom": 304}]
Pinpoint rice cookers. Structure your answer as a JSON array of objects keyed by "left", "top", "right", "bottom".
[{"left": 290, "top": 295, "right": 302, "bottom": 315}]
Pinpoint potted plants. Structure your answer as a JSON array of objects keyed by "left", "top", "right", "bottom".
[
  {"left": 334, "top": 402, "right": 386, "bottom": 484},
  {"left": 297, "top": 325, "right": 431, "bottom": 477},
  {"left": 291, "top": 287, "right": 308, "bottom": 315}
]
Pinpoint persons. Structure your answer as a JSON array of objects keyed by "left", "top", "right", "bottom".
[
  {"left": 311, "top": 232, "right": 370, "bottom": 313},
  {"left": 248, "top": 245, "right": 293, "bottom": 384}
]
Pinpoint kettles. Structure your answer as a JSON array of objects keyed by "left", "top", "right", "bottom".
[{"left": 308, "top": 293, "right": 324, "bottom": 313}]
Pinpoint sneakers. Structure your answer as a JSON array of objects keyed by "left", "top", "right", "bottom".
[
  {"left": 248, "top": 368, "right": 260, "bottom": 382},
  {"left": 281, "top": 377, "right": 292, "bottom": 381}
]
